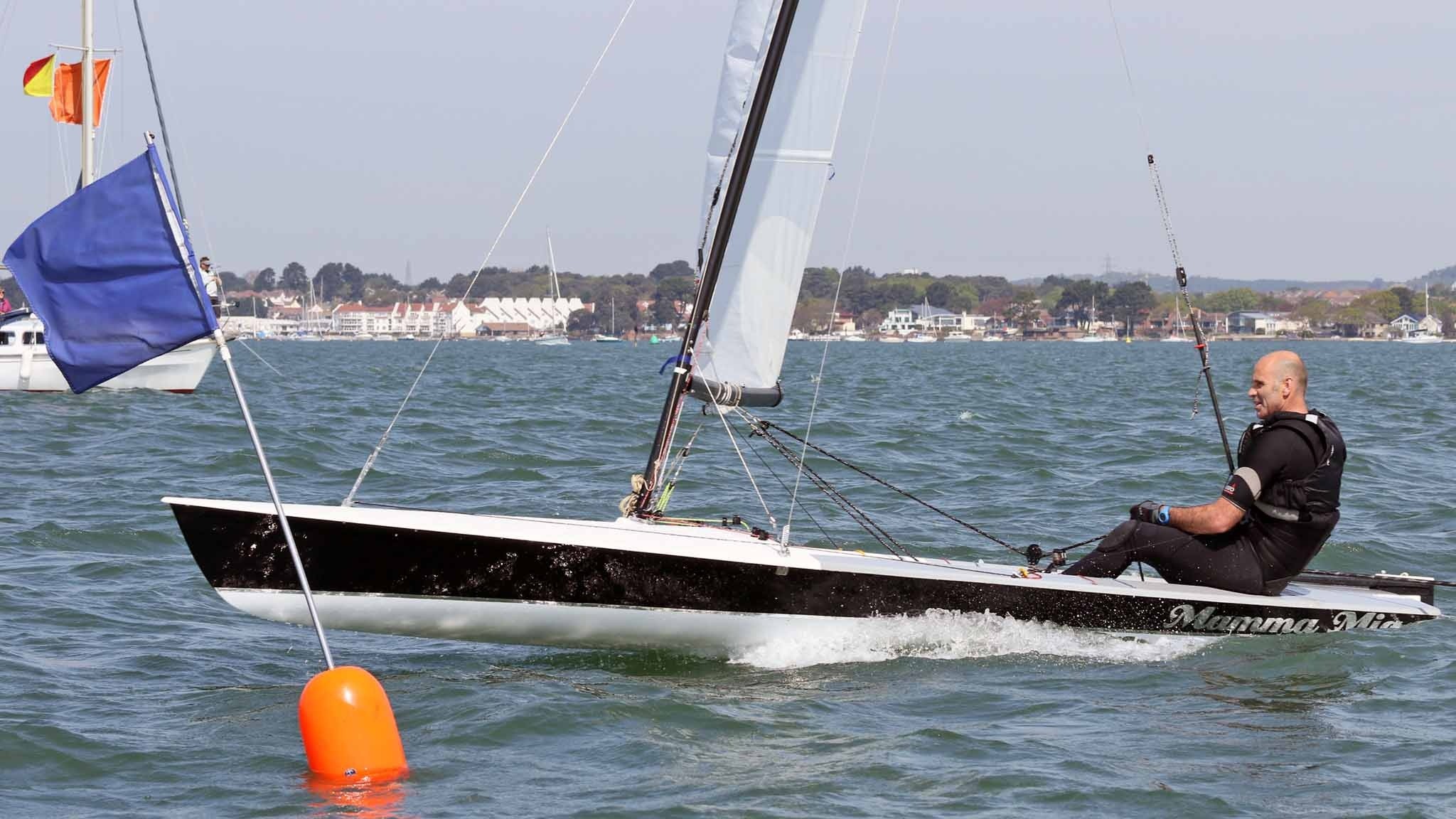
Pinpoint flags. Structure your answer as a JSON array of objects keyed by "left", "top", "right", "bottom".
[
  {"left": 49, "top": 57, "right": 112, "bottom": 127},
  {"left": 2, "top": 141, "right": 221, "bottom": 395},
  {"left": 22, "top": 54, "right": 59, "bottom": 97}
]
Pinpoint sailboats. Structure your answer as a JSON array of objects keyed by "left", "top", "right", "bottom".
[{"left": 0, "top": 0, "right": 256, "bottom": 399}]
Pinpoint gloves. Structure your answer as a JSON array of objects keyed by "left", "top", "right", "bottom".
[{"left": 1130, "top": 500, "right": 1164, "bottom": 524}]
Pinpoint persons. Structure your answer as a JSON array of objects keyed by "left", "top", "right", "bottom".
[
  {"left": 0, "top": 289, "right": 13, "bottom": 318},
  {"left": 196, "top": 256, "right": 221, "bottom": 320},
  {"left": 1061, "top": 350, "right": 1347, "bottom": 594}
]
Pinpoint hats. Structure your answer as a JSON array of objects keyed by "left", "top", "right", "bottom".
[{"left": 201, "top": 261, "right": 213, "bottom": 265}]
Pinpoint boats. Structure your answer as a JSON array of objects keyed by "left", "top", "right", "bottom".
[{"left": 150, "top": 0, "right": 1432, "bottom": 693}]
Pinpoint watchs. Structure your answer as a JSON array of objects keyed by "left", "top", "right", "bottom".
[{"left": 1159, "top": 506, "right": 1171, "bottom": 526}]
